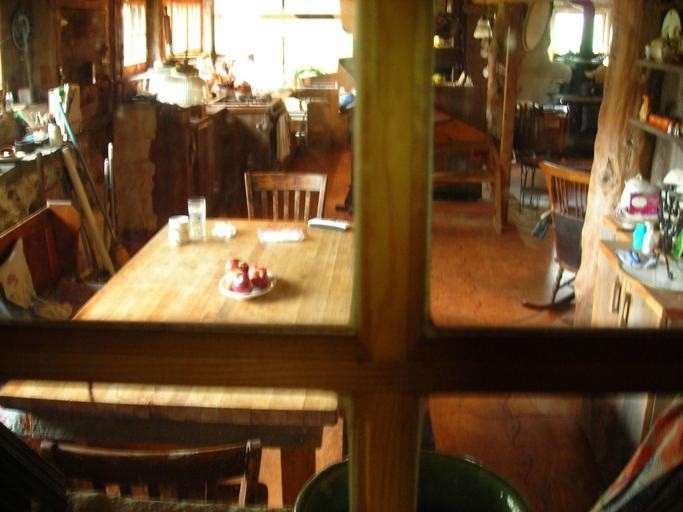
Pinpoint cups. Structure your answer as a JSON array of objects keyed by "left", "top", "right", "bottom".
[
  {"left": 188, "top": 196, "right": 207, "bottom": 245},
  {"left": 168, "top": 215, "right": 189, "bottom": 247}
]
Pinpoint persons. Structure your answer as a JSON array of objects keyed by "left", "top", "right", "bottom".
[{"left": 565, "top": 65, "right": 607, "bottom": 159}]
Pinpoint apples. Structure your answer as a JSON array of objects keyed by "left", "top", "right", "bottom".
[{"left": 225, "top": 260, "right": 269, "bottom": 294}]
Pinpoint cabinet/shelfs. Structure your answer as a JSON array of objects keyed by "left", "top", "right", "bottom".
[
  {"left": 153, "top": 107, "right": 227, "bottom": 224},
  {"left": 211, "top": 95, "right": 295, "bottom": 198},
  {"left": 627, "top": 58, "right": 682, "bottom": 150},
  {"left": 588, "top": 242, "right": 683, "bottom": 454}
]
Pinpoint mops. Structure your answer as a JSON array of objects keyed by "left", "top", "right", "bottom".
[{"left": 57, "top": 101, "right": 131, "bottom": 267}]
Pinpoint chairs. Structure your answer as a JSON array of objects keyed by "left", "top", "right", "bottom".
[
  {"left": 242, "top": 168, "right": 328, "bottom": 221},
  {"left": 0, "top": 208, "right": 98, "bottom": 323},
  {"left": 38, "top": 436, "right": 270, "bottom": 509},
  {"left": 514, "top": 100, "right": 591, "bottom": 312}
]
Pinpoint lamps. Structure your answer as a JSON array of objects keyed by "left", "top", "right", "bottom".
[
  {"left": 473, "top": 0, "right": 492, "bottom": 39},
  {"left": 147, "top": 1, "right": 208, "bottom": 109}
]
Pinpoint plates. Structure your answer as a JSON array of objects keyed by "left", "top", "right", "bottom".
[{"left": 218, "top": 268, "right": 277, "bottom": 301}]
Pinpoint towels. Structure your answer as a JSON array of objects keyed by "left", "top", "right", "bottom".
[{"left": 275, "top": 111, "right": 292, "bottom": 163}]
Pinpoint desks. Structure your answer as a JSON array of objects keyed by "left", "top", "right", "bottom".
[{"left": 0, "top": 215, "right": 354, "bottom": 511}]
